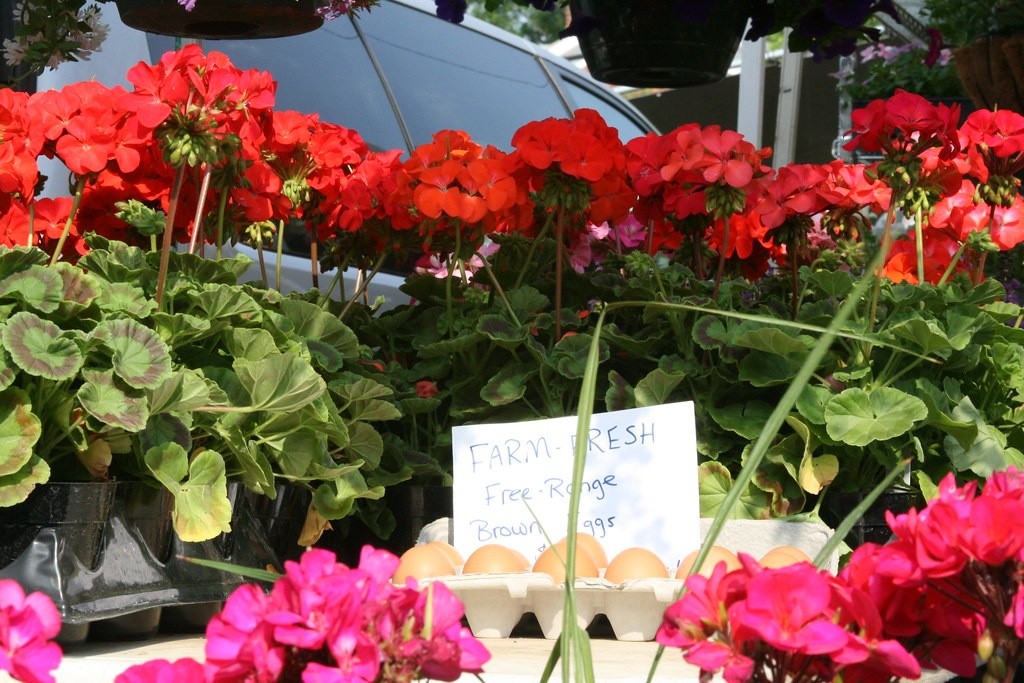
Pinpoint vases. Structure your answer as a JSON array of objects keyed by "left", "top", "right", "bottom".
[
  {"left": 568, "top": 1, "right": 750, "bottom": 87},
  {"left": 0, "top": 479, "right": 302, "bottom": 647},
  {"left": 116, "top": 1, "right": 328, "bottom": 41}
]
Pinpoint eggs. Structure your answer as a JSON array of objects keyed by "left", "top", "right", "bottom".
[{"left": 392, "top": 531, "right": 812, "bottom": 584}]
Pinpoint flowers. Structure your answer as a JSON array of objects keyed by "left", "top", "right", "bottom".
[{"left": 0, "top": 1, "right": 1024, "bottom": 683}]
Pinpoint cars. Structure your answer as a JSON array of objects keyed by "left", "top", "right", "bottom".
[{"left": 35, "top": 0, "right": 670, "bottom": 317}]
